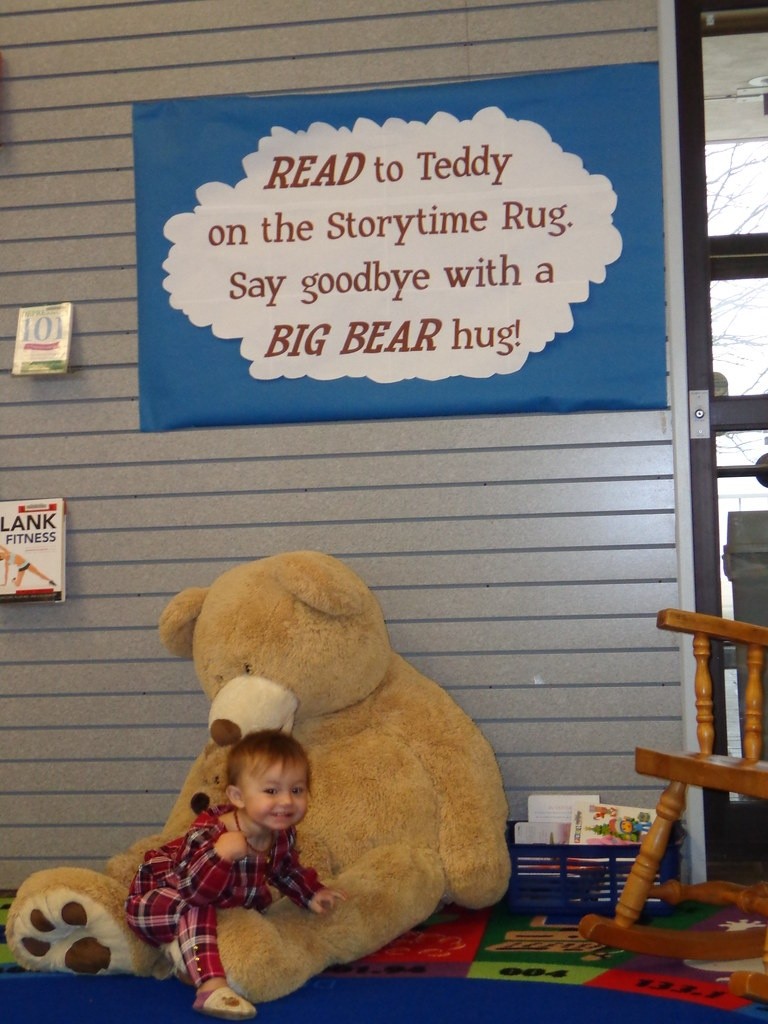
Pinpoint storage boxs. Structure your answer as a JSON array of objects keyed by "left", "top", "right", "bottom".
[{"left": 505, "top": 822, "right": 682, "bottom": 920}]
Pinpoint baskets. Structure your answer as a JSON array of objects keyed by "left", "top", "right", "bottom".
[{"left": 504, "top": 818, "right": 682, "bottom": 921}]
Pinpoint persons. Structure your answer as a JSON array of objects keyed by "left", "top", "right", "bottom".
[{"left": 125, "top": 730, "right": 347, "bottom": 1018}]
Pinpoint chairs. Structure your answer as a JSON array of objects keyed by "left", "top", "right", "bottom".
[{"left": 581, "top": 608, "right": 767, "bottom": 998}]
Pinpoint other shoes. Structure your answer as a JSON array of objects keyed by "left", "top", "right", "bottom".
[{"left": 193, "top": 987, "right": 257, "bottom": 1020}]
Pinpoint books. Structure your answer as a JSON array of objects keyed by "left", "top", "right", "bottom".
[
  {"left": 1, "top": 498, "right": 67, "bottom": 603},
  {"left": 12, "top": 303, "right": 72, "bottom": 375},
  {"left": 515, "top": 794, "right": 661, "bottom": 902}
]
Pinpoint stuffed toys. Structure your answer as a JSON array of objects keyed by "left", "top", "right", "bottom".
[{"left": 6, "top": 550, "right": 511, "bottom": 1002}]
